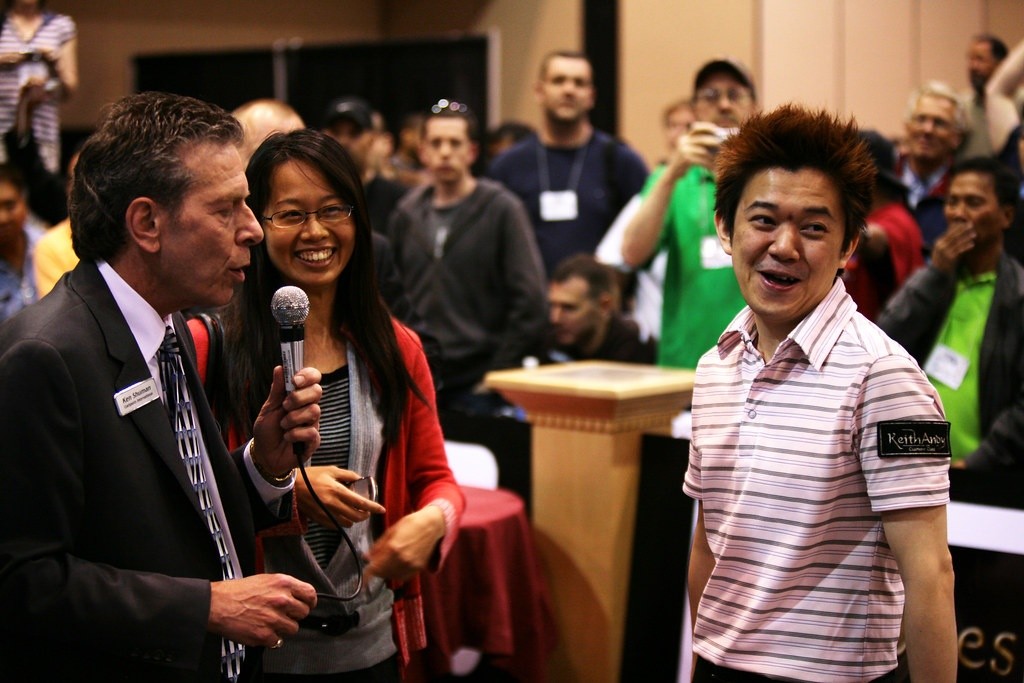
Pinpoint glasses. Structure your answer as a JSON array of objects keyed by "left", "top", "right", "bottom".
[
  {"left": 260, "top": 202, "right": 353, "bottom": 228},
  {"left": 325, "top": 124, "right": 367, "bottom": 138},
  {"left": 694, "top": 89, "right": 747, "bottom": 104}
]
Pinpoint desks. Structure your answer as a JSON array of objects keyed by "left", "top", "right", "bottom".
[{"left": 423, "top": 482, "right": 558, "bottom": 683}]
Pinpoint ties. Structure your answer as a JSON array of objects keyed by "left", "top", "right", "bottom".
[{"left": 155, "top": 326, "right": 246, "bottom": 683}]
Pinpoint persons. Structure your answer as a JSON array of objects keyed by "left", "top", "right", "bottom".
[
  {"left": 0, "top": 0, "right": 659, "bottom": 498},
  {"left": 881, "top": 152, "right": 1024, "bottom": 683},
  {"left": 681, "top": 101, "right": 959, "bottom": 683},
  {"left": 595, "top": 33, "right": 1024, "bottom": 371},
  {"left": 185, "top": 127, "right": 467, "bottom": 683},
  {"left": 0, "top": 93, "right": 322, "bottom": 683}
]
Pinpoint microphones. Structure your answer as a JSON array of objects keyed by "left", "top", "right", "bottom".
[{"left": 271, "top": 286, "right": 311, "bottom": 456}]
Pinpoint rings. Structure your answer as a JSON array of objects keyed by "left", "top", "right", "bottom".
[{"left": 272, "top": 640, "right": 282, "bottom": 649}]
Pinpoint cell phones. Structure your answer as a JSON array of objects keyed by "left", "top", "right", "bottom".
[{"left": 345, "top": 477, "right": 378, "bottom": 502}]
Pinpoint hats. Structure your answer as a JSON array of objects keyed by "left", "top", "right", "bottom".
[{"left": 693, "top": 58, "right": 757, "bottom": 101}]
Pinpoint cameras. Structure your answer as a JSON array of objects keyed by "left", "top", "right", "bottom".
[{"left": 706, "top": 126, "right": 740, "bottom": 157}]
